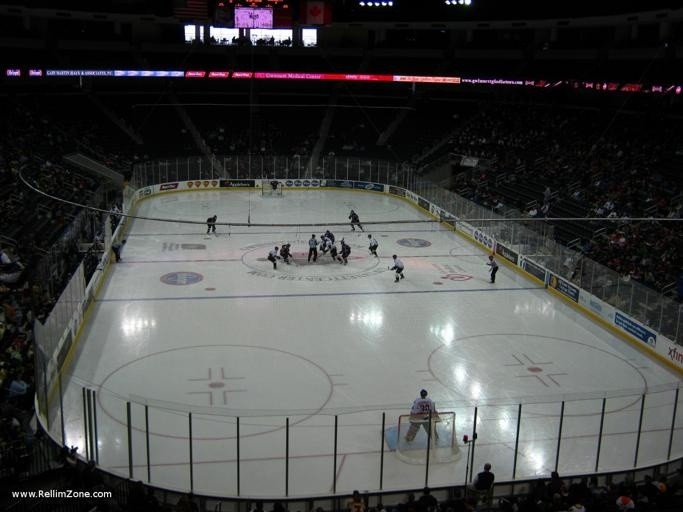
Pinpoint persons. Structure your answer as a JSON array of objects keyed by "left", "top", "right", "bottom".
[
  {"left": 125, "top": 463, "right": 683, "bottom": 511},
  {"left": 266, "top": 229, "right": 352, "bottom": 271},
  {"left": 404, "top": 387, "right": 442, "bottom": 446},
  {"left": 448, "top": 188, "right": 683, "bottom": 348},
  {"left": 0, "top": 191, "right": 127, "bottom": 512},
  {"left": 388, "top": 254, "right": 405, "bottom": 282},
  {"left": 0, "top": 76, "right": 681, "bottom": 189},
  {"left": 366, "top": 234, "right": 378, "bottom": 259},
  {"left": 347, "top": 209, "right": 364, "bottom": 232},
  {"left": 266, "top": 181, "right": 281, "bottom": 195},
  {"left": 205, "top": 214, "right": 216, "bottom": 234}
]
